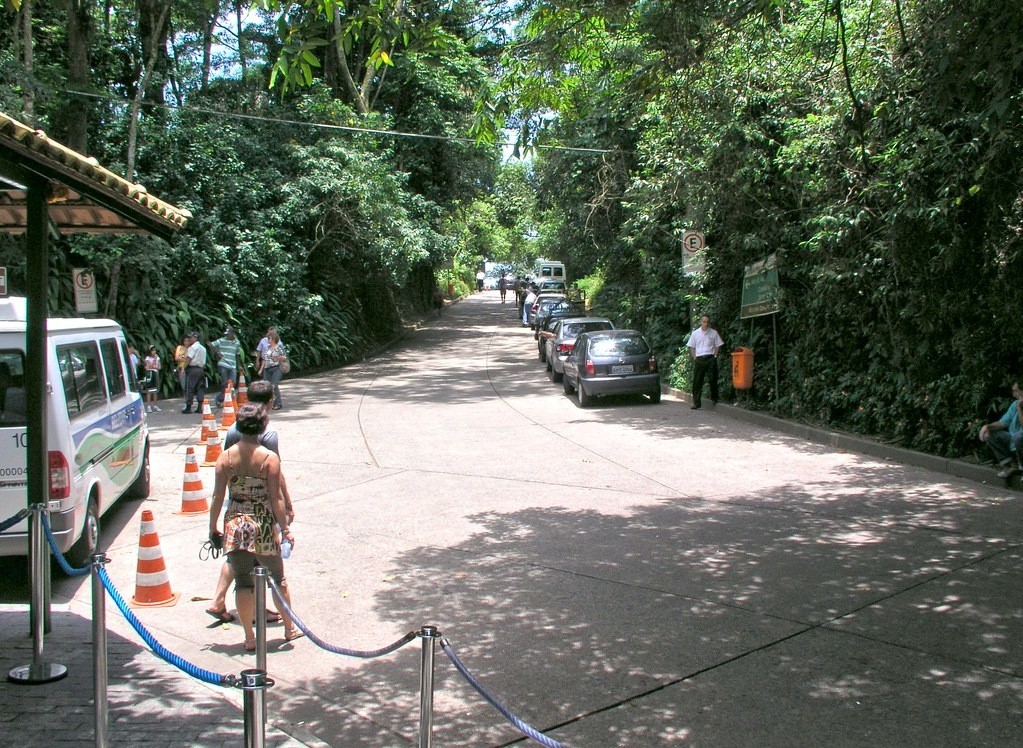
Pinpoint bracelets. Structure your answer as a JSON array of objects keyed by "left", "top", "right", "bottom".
[{"left": 714, "top": 355, "right": 717, "bottom": 358}]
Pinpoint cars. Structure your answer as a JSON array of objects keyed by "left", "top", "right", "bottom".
[
  {"left": 561, "top": 330, "right": 661, "bottom": 407},
  {"left": 484, "top": 261, "right": 617, "bottom": 382}
]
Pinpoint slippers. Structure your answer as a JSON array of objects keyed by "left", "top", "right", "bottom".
[
  {"left": 206, "top": 607, "right": 236, "bottom": 622},
  {"left": 266, "top": 609, "right": 282, "bottom": 622}
]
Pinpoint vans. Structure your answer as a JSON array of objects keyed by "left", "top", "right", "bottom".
[{"left": 0, "top": 296, "right": 160, "bottom": 568}]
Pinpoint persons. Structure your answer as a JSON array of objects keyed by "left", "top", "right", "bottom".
[
  {"left": 180, "top": 333, "right": 217, "bottom": 415},
  {"left": 207, "top": 379, "right": 286, "bottom": 623},
  {"left": 146, "top": 347, "right": 163, "bottom": 412},
  {"left": 254, "top": 326, "right": 284, "bottom": 371},
  {"left": 207, "top": 403, "right": 307, "bottom": 650},
  {"left": 513, "top": 275, "right": 540, "bottom": 319},
  {"left": 497, "top": 274, "right": 508, "bottom": 304},
  {"left": 257, "top": 332, "right": 284, "bottom": 409},
  {"left": 130, "top": 345, "right": 140, "bottom": 379},
  {"left": 571, "top": 283, "right": 581, "bottom": 301},
  {"left": 176, "top": 336, "right": 190, "bottom": 390},
  {"left": 207, "top": 327, "right": 246, "bottom": 407},
  {"left": 523, "top": 291, "right": 537, "bottom": 327},
  {"left": 686, "top": 314, "right": 725, "bottom": 410},
  {"left": 476, "top": 269, "right": 486, "bottom": 293},
  {"left": 977, "top": 381, "right": 1023, "bottom": 483}
]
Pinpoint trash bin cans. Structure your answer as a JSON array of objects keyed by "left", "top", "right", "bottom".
[{"left": 730, "top": 346, "right": 755, "bottom": 390}]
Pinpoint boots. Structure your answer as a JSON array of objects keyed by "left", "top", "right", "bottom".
[
  {"left": 182, "top": 404, "right": 191, "bottom": 414},
  {"left": 194, "top": 402, "right": 203, "bottom": 413}
]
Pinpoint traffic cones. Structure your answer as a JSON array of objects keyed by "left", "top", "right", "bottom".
[
  {"left": 170, "top": 447, "right": 212, "bottom": 516},
  {"left": 200, "top": 414, "right": 222, "bottom": 467},
  {"left": 237, "top": 370, "right": 247, "bottom": 407},
  {"left": 196, "top": 400, "right": 212, "bottom": 445},
  {"left": 219, "top": 379, "right": 239, "bottom": 430},
  {"left": 130, "top": 511, "right": 180, "bottom": 607}
]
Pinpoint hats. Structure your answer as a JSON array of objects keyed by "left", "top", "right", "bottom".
[
  {"left": 224, "top": 327, "right": 233, "bottom": 335},
  {"left": 187, "top": 332, "right": 200, "bottom": 339}
]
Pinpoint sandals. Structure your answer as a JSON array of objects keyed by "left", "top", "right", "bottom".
[
  {"left": 244, "top": 639, "right": 256, "bottom": 651},
  {"left": 286, "top": 628, "right": 304, "bottom": 640}
]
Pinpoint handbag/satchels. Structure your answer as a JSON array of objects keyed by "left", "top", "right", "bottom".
[
  {"left": 279, "top": 358, "right": 291, "bottom": 374},
  {"left": 136, "top": 358, "right": 146, "bottom": 380}
]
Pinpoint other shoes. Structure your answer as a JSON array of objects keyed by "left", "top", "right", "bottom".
[
  {"left": 154, "top": 405, "right": 162, "bottom": 412},
  {"left": 712, "top": 399, "right": 718, "bottom": 405},
  {"left": 147, "top": 406, "right": 152, "bottom": 413},
  {"left": 274, "top": 405, "right": 281, "bottom": 409},
  {"left": 997, "top": 464, "right": 1019, "bottom": 478},
  {"left": 691, "top": 404, "right": 701, "bottom": 409}
]
